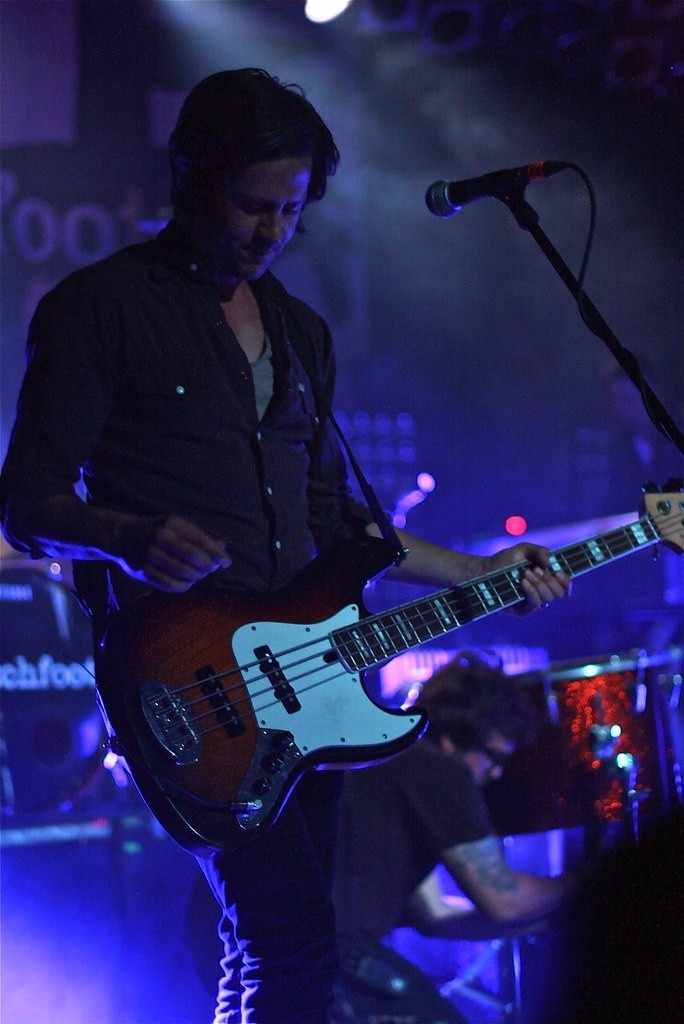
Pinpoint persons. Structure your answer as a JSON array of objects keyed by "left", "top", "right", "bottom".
[
  {"left": 1, "top": 65, "right": 572, "bottom": 1024},
  {"left": 185, "top": 649, "right": 584, "bottom": 1024}
]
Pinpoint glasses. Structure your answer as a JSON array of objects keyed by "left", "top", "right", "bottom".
[{"left": 476, "top": 743, "right": 514, "bottom": 772}]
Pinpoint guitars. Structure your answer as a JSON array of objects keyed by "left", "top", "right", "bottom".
[{"left": 89, "top": 476, "right": 683, "bottom": 860}]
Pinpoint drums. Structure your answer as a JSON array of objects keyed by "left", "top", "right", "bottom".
[{"left": 477, "top": 648, "right": 683, "bottom": 834}]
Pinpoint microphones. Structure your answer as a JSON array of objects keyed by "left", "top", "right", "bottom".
[{"left": 426, "top": 160, "right": 565, "bottom": 219}]
